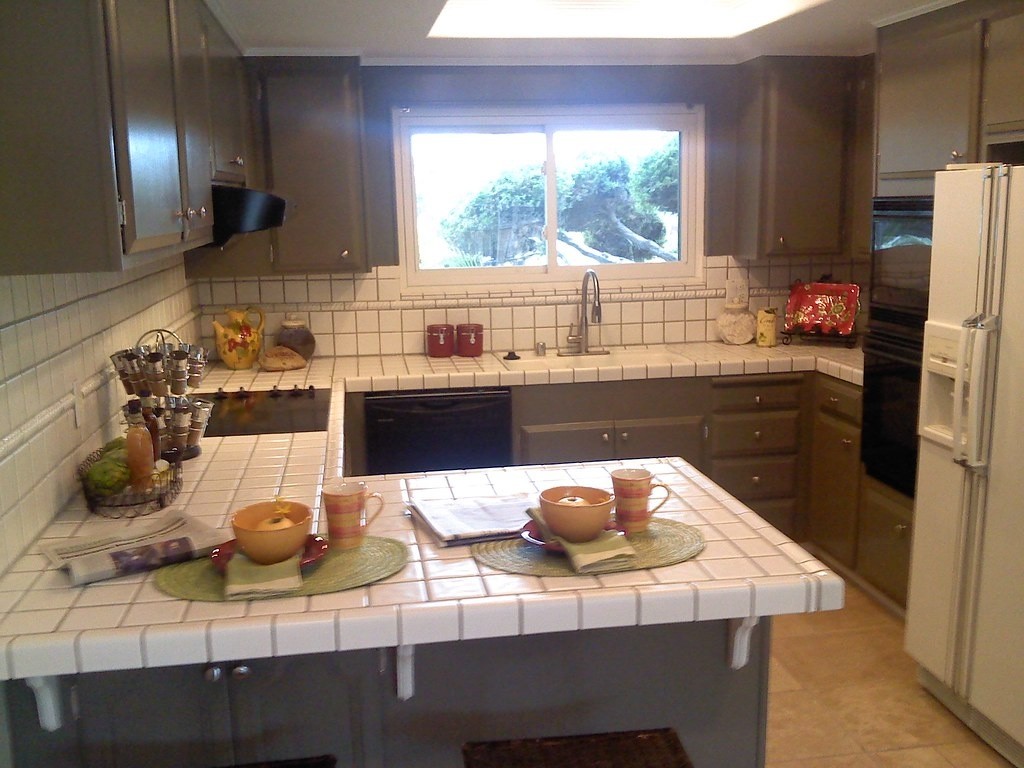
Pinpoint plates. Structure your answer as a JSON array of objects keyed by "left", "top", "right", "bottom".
[
  {"left": 784, "top": 279, "right": 859, "bottom": 336},
  {"left": 208, "top": 534, "right": 330, "bottom": 573},
  {"left": 521, "top": 517, "right": 629, "bottom": 554}
]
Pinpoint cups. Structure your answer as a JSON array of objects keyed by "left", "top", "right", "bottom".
[
  {"left": 612, "top": 469, "right": 671, "bottom": 533},
  {"left": 322, "top": 480, "right": 385, "bottom": 549}
]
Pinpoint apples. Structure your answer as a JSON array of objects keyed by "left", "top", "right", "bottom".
[
  {"left": 557, "top": 495, "right": 590, "bottom": 506},
  {"left": 82, "top": 437, "right": 133, "bottom": 496},
  {"left": 253, "top": 517, "right": 295, "bottom": 531}
]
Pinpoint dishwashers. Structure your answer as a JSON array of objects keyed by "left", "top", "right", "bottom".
[{"left": 363, "top": 390, "right": 513, "bottom": 473}]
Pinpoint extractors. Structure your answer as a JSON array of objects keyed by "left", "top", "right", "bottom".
[{"left": 211, "top": 184, "right": 286, "bottom": 250}]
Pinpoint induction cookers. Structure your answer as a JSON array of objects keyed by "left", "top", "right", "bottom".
[{"left": 187, "top": 384, "right": 332, "bottom": 436}]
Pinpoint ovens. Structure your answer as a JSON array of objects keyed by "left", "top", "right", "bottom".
[{"left": 860, "top": 195, "right": 933, "bottom": 502}]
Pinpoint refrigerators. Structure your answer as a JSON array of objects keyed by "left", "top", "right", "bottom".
[{"left": 898, "top": 163, "right": 1024, "bottom": 766}]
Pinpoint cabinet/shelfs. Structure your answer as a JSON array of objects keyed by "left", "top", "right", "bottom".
[
  {"left": 204, "top": 5, "right": 249, "bottom": 187},
  {"left": 736, "top": 57, "right": 848, "bottom": 264},
  {"left": 873, "top": 12, "right": 984, "bottom": 198},
  {"left": 713, "top": 374, "right": 802, "bottom": 543},
  {"left": 512, "top": 377, "right": 709, "bottom": 473},
  {"left": 0, "top": 0, "right": 213, "bottom": 276},
  {"left": 805, "top": 373, "right": 861, "bottom": 570},
  {"left": 858, "top": 474, "right": 916, "bottom": 611},
  {"left": 264, "top": 56, "right": 373, "bottom": 276}
]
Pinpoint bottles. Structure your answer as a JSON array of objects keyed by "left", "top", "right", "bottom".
[
  {"left": 715, "top": 302, "right": 756, "bottom": 344},
  {"left": 124, "top": 399, "right": 155, "bottom": 484},
  {"left": 276, "top": 318, "right": 316, "bottom": 361},
  {"left": 110, "top": 342, "right": 214, "bottom": 450},
  {"left": 428, "top": 324, "right": 454, "bottom": 357},
  {"left": 140, "top": 398, "right": 159, "bottom": 462},
  {"left": 457, "top": 324, "right": 483, "bottom": 356}
]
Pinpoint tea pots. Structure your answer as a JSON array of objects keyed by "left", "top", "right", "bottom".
[{"left": 211, "top": 307, "right": 265, "bottom": 369}]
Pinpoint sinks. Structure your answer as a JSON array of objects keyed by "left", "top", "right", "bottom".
[{"left": 507, "top": 352, "right": 691, "bottom": 372}]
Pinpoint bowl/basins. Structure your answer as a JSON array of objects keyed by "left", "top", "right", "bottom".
[
  {"left": 230, "top": 500, "right": 314, "bottom": 564},
  {"left": 539, "top": 486, "right": 615, "bottom": 542}
]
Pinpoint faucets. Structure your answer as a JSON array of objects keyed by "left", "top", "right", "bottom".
[{"left": 566, "top": 269, "right": 603, "bottom": 355}]
